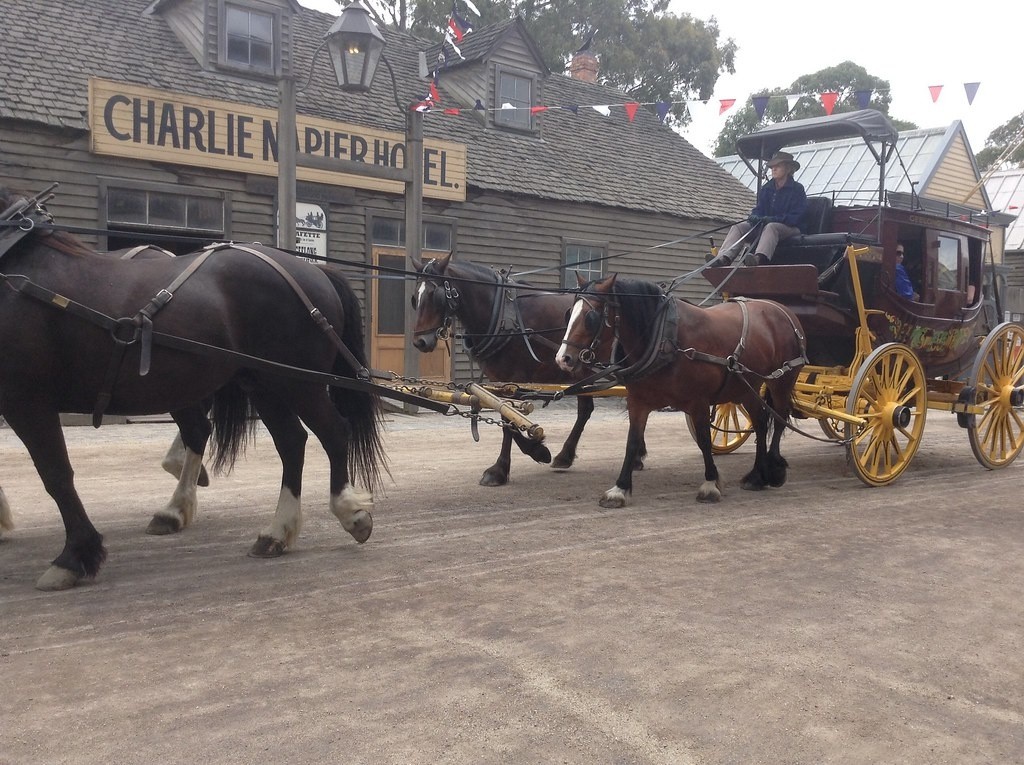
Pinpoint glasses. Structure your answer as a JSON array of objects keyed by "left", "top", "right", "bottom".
[{"left": 896, "top": 251, "right": 905, "bottom": 256}]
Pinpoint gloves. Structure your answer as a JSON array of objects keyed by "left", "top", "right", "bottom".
[{"left": 748, "top": 214, "right": 776, "bottom": 226}]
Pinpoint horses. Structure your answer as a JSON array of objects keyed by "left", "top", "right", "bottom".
[
  {"left": 551, "top": 270, "right": 808, "bottom": 511},
  {"left": 92, "top": 243, "right": 209, "bottom": 536},
  {"left": 0, "top": 186, "right": 376, "bottom": 592},
  {"left": 407, "top": 248, "right": 648, "bottom": 486}
]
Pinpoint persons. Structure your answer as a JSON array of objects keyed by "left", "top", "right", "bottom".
[
  {"left": 896, "top": 245, "right": 920, "bottom": 301},
  {"left": 705, "top": 152, "right": 807, "bottom": 267},
  {"left": 968, "top": 261, "right": 979, "bottom": 308}
]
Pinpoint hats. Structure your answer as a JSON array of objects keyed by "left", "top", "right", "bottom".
[{"left": 765, "top": 152, "right": 800, "bottom": 173}]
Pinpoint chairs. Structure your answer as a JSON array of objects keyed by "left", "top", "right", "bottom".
[{"left": 742, "top": 196, "right": 831, "bottom": 266}]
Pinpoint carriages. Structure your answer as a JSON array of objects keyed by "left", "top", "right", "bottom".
[{"left": 1, "top": 106, "right": 1024, "bottom": 592}]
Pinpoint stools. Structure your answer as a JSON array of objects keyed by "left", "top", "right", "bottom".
[{"left": 801, "top": 232, "right": 884, "bottom": 305}]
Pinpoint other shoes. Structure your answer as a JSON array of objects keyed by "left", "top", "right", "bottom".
[
  {"left": 744, "top": 254, "right": 761, "bottom": 266},
  {"left": 705, "top": 253, "right": 731, "bottom": 268}
]
